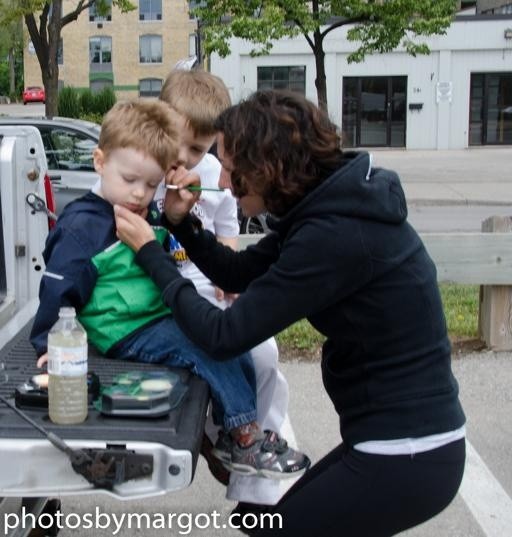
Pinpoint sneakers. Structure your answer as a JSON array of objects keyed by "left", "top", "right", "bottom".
[{"left": 210, "top": 428, "right": 311, "bottom": 476}]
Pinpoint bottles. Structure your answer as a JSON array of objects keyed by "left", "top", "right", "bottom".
[{"left": 47, "top": 306, "right": 88, "bottom": 424}]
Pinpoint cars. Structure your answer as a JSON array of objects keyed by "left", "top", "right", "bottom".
[
  {"left": 22, "top": 86, "right": 46, "bottom": 105},
  {"left": 0, "top": 115, "right": 101, "bottom": 224}
]
